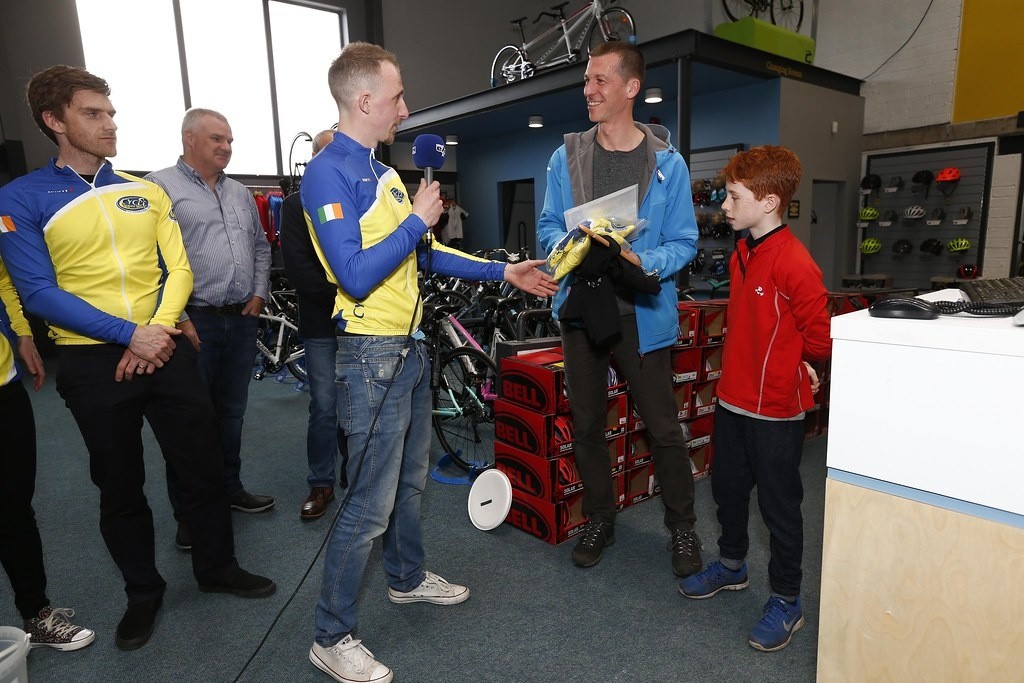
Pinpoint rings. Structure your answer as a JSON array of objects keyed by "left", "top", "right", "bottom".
[{"left": 138, "top": 365, "right": 146, "bottom": 370}]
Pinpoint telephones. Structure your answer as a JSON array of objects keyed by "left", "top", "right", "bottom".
[{"left": 867, "top": 292, "right": 939, "bottom": 320}]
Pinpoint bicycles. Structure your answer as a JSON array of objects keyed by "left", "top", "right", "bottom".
[
  {"left": 490, "top": 0, "right": 638, "bottom": 89},
  {"left": 248, "top": 239, "right": 737, "bottom": 473},
  {"left": 721, "top": 0, "right": 805, "bottom": 34}
]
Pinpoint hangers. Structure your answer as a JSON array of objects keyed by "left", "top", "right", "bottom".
[{"left": 252, "top": 184, "right": 284, "bottom": 198}]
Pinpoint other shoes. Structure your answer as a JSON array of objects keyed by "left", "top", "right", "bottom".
[
  {"left": 114, "top": 598, "right": 163, "bottom": 650},
  {"left": 197, "top": 569, "right": 276, "bottom": 598},
  {"left": 299, "top": 487, "right": 335, "bottom": 519}
]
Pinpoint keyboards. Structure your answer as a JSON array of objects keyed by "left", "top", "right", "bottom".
[{"left": 959, "top": 277, "right": 1024, "bottom": 309}]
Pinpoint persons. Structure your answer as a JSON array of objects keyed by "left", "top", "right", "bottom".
[
  {"left": 1, "top": 66, "right": 279, "bottom": 653},
  {"left": 678, "top": 146, "right": 831, "bottom": 654},
  {"left": 297, "top": 40, "right": 560, "bottom": 683},
  {"left": 141, "top": 109, "right": 277, "bottom": 552},
  {"left": 0, "top": 257, "right": 97, "bottom": 650},
  {"left": 280, "top": 129, "right": 349, "bottom": 521},
  {"left": 535, "top": 40, "right": 703, "bottom": 578}
]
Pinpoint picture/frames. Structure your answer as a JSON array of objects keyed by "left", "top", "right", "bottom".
[{"left": 788, "top": 200, "right": 800, "bottom": 219}]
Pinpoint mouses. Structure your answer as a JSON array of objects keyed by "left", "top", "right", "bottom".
[{"left": 869, "top": 294, "right": 942, "bottom": 319}]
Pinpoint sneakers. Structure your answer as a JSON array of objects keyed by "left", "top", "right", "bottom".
[
  {"left": 308, "top": 634, "right": 394, "bottom": 683},
  {"left": 228, "top": 490, "right": 275, "bottom": 513},
  {"left": 175, "top": 521, "right": 192, "bottom": 549},
  {"left": 23, "top": 605, "right": 95, "bottom": 651},
  {"left": 387, "top": 571, "right": 470, "bottom": 605},
  {"left": 747, "top": 596, "right": 805, "bottom": 652},
  {"left": 676, "top": 560, "right": 749, "bottom": 599},
  {"left": 666, "top": 528, "right": 704, "bottom": 576},
  {"left": 571, "top": 520, "right": 615, "bottom": 567}
]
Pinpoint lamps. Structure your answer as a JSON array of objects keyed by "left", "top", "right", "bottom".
[
  {"left": 445, "top": 135, "right": 459, "bottom": 146},
  {"left": 528, "top": 116, "right": 544, "bottom": 128},
  {"left": 644, "top": 87, "right": 663, "bottom": 104}
]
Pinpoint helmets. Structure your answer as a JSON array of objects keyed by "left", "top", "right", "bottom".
[
  {"left": 859, "top": 238, "right": 882, "bottom": 254},
  {"left": 919, "top": 238, "right": 943, "bottom": 254},
  {"left": 903, "top": 205, "right": 926, "bottom": 218},
  {"left": 911, "top": 170, "right": 934, "bottom": 183},
  {"left": 860, "top": 175, "right": 881, "bottom": 189},
  {"left": 957, "top": 263, "right": 977, "bottom": 279},
  {"left": 891, "top": 239, "right": 912, "bottom": 253},
  {"left": 947, "top": 238, "right": 971, "bottom": 251},
  {"left": 859, "top": 207, "right": 880, "bottom": 221},
  {"left": 935, "top": 167, "right": 961, "bottom": 181}
]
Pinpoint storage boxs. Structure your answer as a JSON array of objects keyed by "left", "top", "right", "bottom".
[{"left": 492, "top": 290, "right": 870, "bottom": 547}]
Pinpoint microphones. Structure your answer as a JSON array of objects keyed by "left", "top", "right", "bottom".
[{"left": 412, "top": 134, "right": 446, "bottom": 248}]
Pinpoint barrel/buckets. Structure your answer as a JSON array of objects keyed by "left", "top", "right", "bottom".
[{"left": 0, "top": 625, "right": 32, "bottom": 683}]
